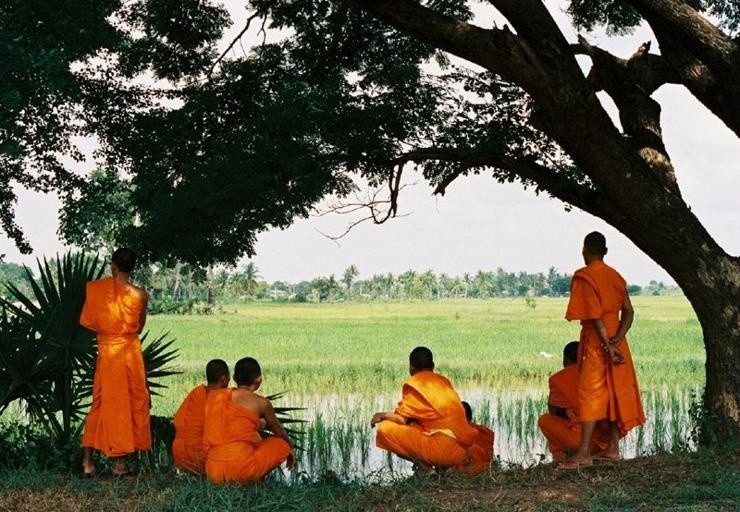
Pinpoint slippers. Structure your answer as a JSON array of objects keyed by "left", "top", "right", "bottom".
[
  {"left": 556, "top": 455, "right": 593, "bottom": 469},
  {"left": 413, "top": 468, "right": 437, "bottom": 476},
  {"left": 83, "top": 464, "right": 97, "bottom": 476},
  {"left": 113, "top": 464, "right": 132, "bottom": 475},
  {"left": 593, "top": 451, "right": 620, "bottom": 461}
]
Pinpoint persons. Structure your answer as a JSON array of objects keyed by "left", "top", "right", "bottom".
[
  {"left": 204, "top": 357, "right": 294, "bottom": 486},
  {"left": 460, "top": 401, "right": 496, "bottom": 470},
  {"left": 539, "top": 342, "right": 609, "bottom": 462},
  {"left": 557, "top": 231, "right": 646, "bottom": 468},
  {"left": 80, "top": 249, "right": 153, "bottom": 474},
  {"left": 173, "top": 360, "right": 232, "bottom": 474},
  {"left": 371, "top": 348, "right": 478, "bottom": 474}
]
